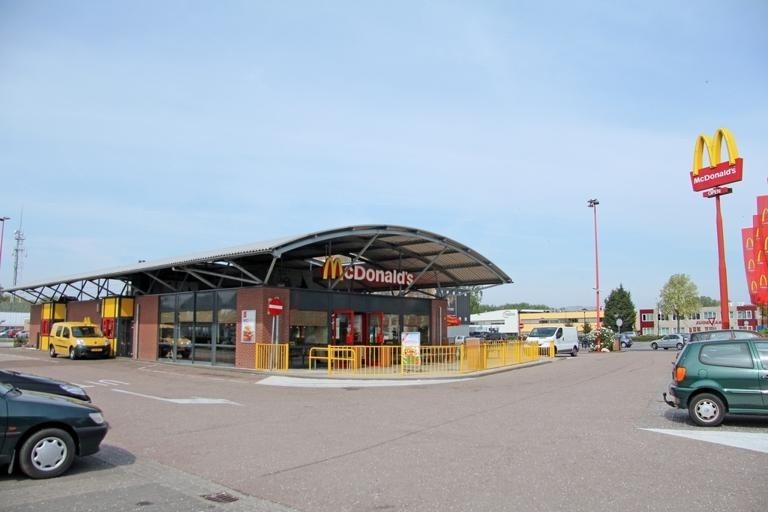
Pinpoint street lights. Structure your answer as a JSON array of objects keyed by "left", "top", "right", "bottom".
[
  {"left": 0, "top": 217, "right": 10, "bottom": 262},
  {"left": 582, "top": 309, "right": 587, "bottom": 324},
  {"left": 588, "top": 198, "right": 601, "bottom": 352}
]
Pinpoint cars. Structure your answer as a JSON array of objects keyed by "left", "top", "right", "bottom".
[
  {"left": 651, "top": 334, "right": 685, "bottom": 350},
  {"left": 0, "top": 329, "right": 30, "bottom": 338},
  {"left": 663, "top": 330, "right": 768, "bottom": 427},
  {"left": 0, "top": 370, "right": 108, "bottom": 479},
  {"left": 582, "top": 327, "right": 638, "bottom": 347}
]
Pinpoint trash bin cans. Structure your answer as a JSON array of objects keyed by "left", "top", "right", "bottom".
[
  {"left": 14, "top": 337, "right": 21, "bottom": 347},
  {"left": 613, "top": 336, "right": 621, "bottom": 351},
  {"left": 466, "top": 338, "right": 484, "bottom": 369},
  {"left": 381, "top": 347, "right": 392, "bottom": 367},
  {"left": 350, "top": 346, "right": 362, "bottom": 368}
]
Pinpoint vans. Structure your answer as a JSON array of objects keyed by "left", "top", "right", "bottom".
[
  {"left": 49, "top": 322, "right": 111, "bottom": 359},
  {"left": 525, "top": 326, "right": 579, "bottom": 356},
  {"left": 159, "top": 324, "right": 192, "bottom": 358}
]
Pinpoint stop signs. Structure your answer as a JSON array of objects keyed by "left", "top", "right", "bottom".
[
  {"left": 268, "top": 299, "right": 284, "bottom": 316},
  {"left": 519, "top": 324, "right": 524, "bottom": 329}
]
